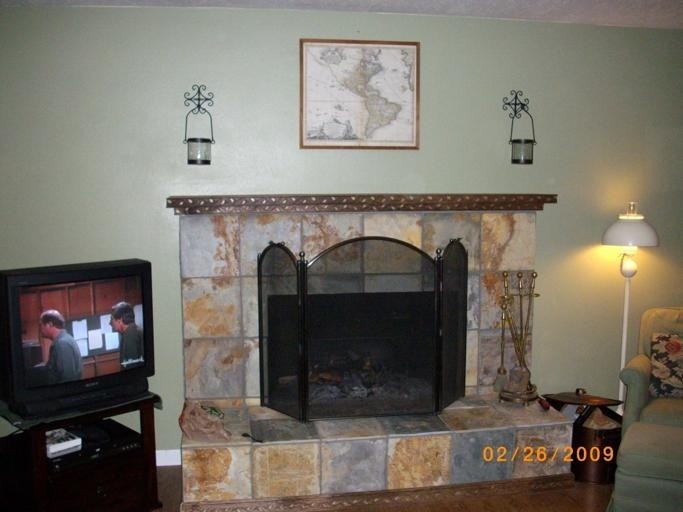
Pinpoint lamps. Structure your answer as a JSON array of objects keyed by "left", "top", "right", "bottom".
[
  {"left": 183, "top": 84, "right": 215, "bottom": 165},
  {"left": 601, "top": 202, "right": 659, "bottom": 417},
  {"left": 502, "top": 90, "right": 537, "bottom": 164}
]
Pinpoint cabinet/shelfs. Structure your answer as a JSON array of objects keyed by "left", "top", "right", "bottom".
[{"left": 0, "top": 391, "right": 164, "bottom": 510}]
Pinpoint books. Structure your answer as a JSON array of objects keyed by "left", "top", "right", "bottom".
[{"left": 47, "top": 428, "right": 82, "bottom": 460}]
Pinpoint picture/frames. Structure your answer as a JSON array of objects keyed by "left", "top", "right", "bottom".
[{"left": 299, "top": 38, "right": 421, "bottom": 150}]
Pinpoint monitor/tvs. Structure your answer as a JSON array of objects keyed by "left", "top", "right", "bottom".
[{"left": 0, "top": 258, "right": 156, "bottom": 417}]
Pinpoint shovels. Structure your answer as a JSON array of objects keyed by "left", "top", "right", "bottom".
[{"left": 503, "top": 272, "right": 537, "bottom": 393}]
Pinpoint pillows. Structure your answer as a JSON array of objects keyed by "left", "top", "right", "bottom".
[{"left": 648, "top": 332, "right": 683, "bottom": 399}]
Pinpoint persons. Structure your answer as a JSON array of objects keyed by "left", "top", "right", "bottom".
[
  {"left": 38, "top": 308, "right": 83, "bottom": 386},
  {"left": 109, "top": 302, "right": 146, "bottom": 371}
]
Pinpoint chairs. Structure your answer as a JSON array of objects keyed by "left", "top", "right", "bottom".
[{"left": 604, "top": 307, "right": 683, "bottom": 512}]
